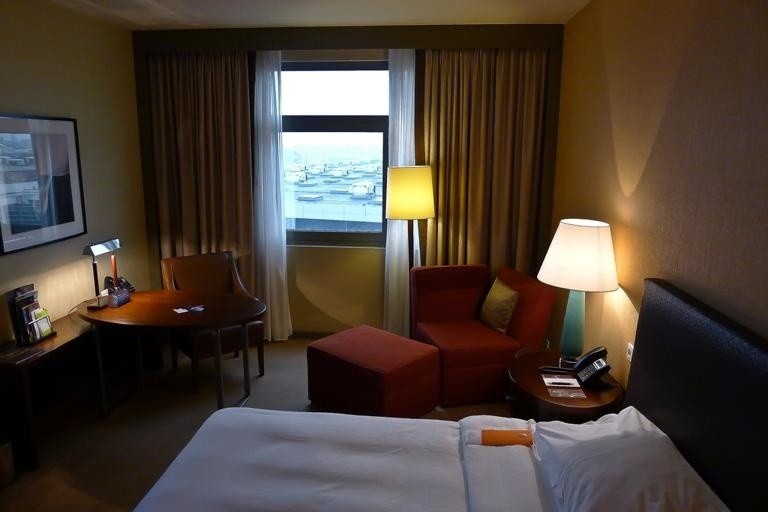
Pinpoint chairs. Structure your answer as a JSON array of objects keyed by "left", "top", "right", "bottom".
[
  {"left": 160, "top": 250, "right": 265, "bottom": 394},
  {"left": 409, "top": 264, "right": 557, "bottom": 408}
]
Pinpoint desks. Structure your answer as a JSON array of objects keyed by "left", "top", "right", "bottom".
[
  {"left": 78, "top": 289, "right": 267, "bottom": 410},
  {"left": 1, "top": 312, "right": 163, "bottom": 472}
]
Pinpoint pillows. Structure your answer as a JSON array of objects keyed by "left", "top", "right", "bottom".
[
  {"left": 528, "top": 405, "right": 731, "bottom": 512},
  {"left": 480, "top": 277, "right": 520, "bottom": 336}
]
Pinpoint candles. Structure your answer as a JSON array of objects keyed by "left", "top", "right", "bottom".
[{"left": 111, "top": 255, "right": 118, "bottom": 286}]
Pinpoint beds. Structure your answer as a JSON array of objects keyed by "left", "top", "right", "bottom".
[{"left": 130, "top": 279, "right": 767, "bottom": 512}]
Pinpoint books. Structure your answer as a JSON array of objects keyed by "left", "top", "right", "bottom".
[{"left": 5, "top": 282, "right": 52, "bottom": 344}]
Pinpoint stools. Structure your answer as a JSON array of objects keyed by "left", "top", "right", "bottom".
[{"left": 307, "top": 324, "right": 440, "bottom": 418}]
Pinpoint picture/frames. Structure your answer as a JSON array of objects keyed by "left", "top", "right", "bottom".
[{"left": 0, "top": 113, "right": 88, "bottom": 256}]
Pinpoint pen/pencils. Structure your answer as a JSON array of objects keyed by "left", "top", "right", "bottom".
[{"left": 547, "top": 382, "right": 573, "bottom": 385}]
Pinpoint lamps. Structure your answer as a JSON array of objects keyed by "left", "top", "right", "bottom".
[
  {"left": 385, "top": 165, "right": 436, "bottom": 339},
  {"left": 537, "top": 218, "right": 619, "bottom": 369},
  {"left": 82, "top": 237, "right": 122, "bottom": 311}
]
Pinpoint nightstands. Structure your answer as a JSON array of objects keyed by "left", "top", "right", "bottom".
[{"left": 505, "top": 351, "right": 625, "bottom": 424}]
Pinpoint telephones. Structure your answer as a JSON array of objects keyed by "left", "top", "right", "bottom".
[
  {"left": 574, "top": 346, "right": 611, "bottom": 384},
  {"left": 104, "top": 277, "right": 135, "bottom": 294}
]
